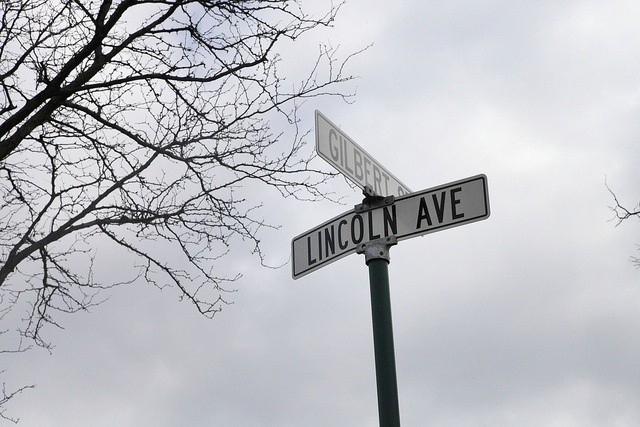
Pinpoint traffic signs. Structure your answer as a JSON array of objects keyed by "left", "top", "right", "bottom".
[
  {"left": 291, "top": 173, "right": 491, "bottom": 279},
  {"left": 315, "top": 110, "right": 415, "bottom": 197}
]
